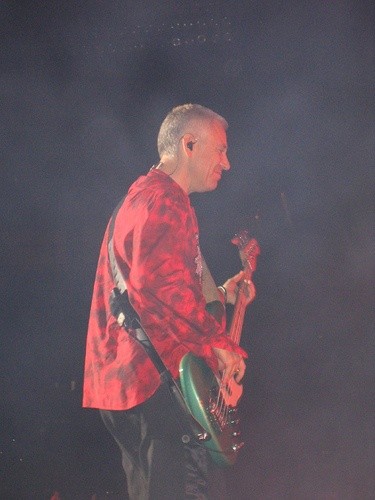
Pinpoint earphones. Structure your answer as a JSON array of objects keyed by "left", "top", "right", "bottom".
[{"left": 187, "top": 141, "right": 193, "bottom": 150}]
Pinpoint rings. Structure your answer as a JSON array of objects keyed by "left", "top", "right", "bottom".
[{"left": 235, "top": 369, "right": 240, "bottom": 372}]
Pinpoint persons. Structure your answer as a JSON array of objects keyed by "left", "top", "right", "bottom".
[{"left": 81, "top": 103, "right": 255, "bottom": 499}]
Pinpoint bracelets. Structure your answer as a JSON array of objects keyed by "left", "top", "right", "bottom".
[{"left": 217, "top": 286, "right": 228, "bottom": 305}]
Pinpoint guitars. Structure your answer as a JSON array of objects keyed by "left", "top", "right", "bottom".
[{"left": 177, "top": 227, "right": 261, "bottom": 473}]
